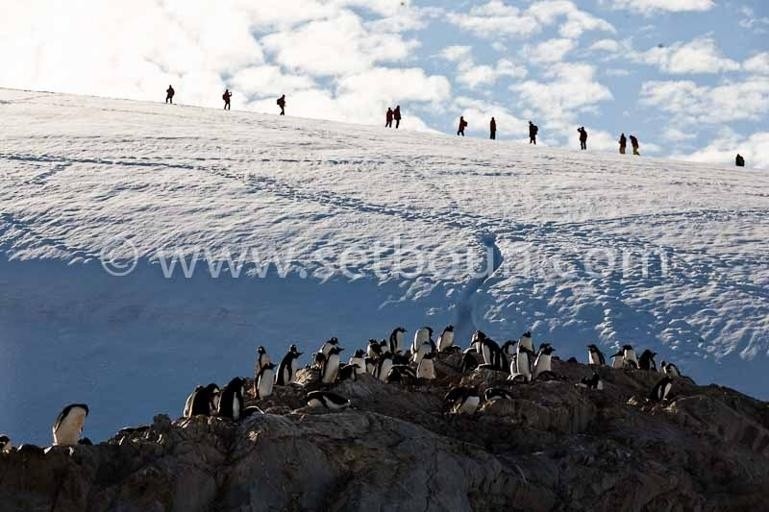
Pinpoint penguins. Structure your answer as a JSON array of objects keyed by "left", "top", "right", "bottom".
[
  {"left": 183, "top": 377, "right": 248, "bottom": 420},
  {"left": 586, "top": 343, "right": 605, "bottom": 367},
  {"left": 51, "top": 401, "right": 89, "bottom": 449},
  {"left": 609, "top": 343, "right": 658, "bottom": 370},
  {"left": 660, "top": 360, "right": 679, "bottom": 373},
  {"left": 651, "top": 376, "right": 674, "bottom": 404},
  {"left": 0, "top": 431, "right": 13, "bottom": 456},
  {"left": 589, "top": 373, "right": 604, "bottom": 392},
  {"left": 249, "top": 343, "right": 306, "bottom": 399},
  {"left": 305, "top": 322, "right": 558, "bottom": 420}
]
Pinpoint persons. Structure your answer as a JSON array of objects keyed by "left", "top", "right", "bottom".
[
  {"left": 577, "top": 127, "right": 587, "bottom": 150},
  {"left": 280, "top": 94, "right": 286, "bottom": 116},
  {"left": 223, "top": 89, "right": 232, "bottom": 109},
  {"left": 489, "top": 117, "right": 496, "bottom": 140},
  {"left": 385, "top": 107, "right": 394, "bottom": 127},
  {"left": 529, "top": 121, "right": 536, "bottom": 144},
  {"left": 165, "top": 84, "right": 176, "bottom": 103},
  {"left": 619, "top": 133, "right": 627, "bottom": 153},
  {"left": 457, "top": 116, "right": 467, "bottom": 136},
  {"left": 735, "top": 153, "right": 745, "bottom": 167},
  {"left": 630, "top": 134, "right": 640, "bottom": 155},
  {"left": 395, "top": 105, "right": 402, "bottom": 128}
]
